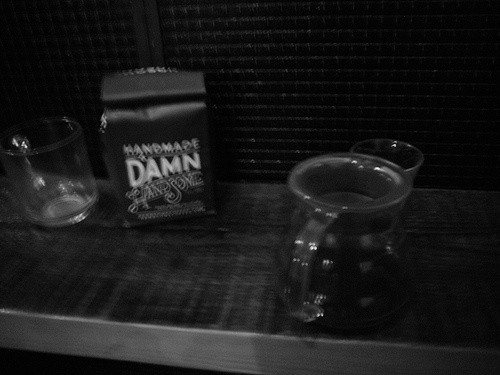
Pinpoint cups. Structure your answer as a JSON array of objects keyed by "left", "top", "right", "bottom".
[
  {"left": 2, "top": 116, "right": 97, "bottom": 228},
  {"left": 287, "top": 153, "right": 411, "bottom": 332},
  {"left": 351, "top": 138, "right": 425, "bottom": 182}
]
